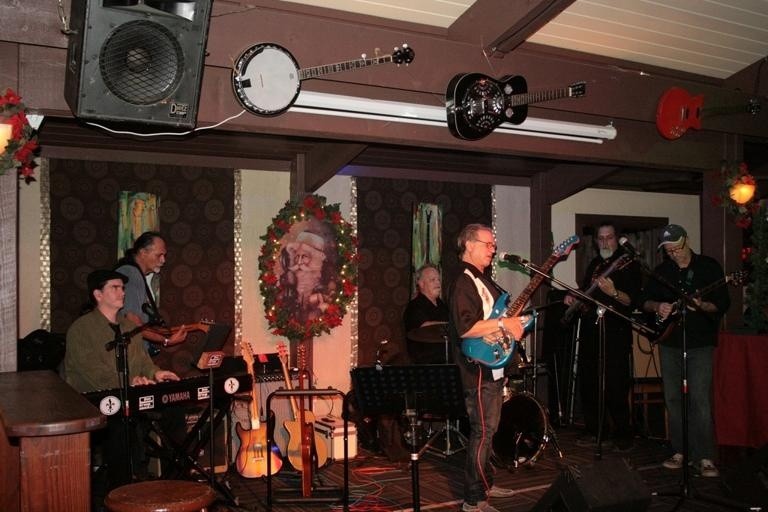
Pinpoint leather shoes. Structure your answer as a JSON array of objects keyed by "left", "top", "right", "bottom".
[
  {"left": 462, "top": 501, "right": 500, "bottom": 512},
  {"left": 485, "top": 487, "right": 513, "bottom": 498}
]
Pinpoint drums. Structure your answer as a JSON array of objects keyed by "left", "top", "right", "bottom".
[{"left": 492, "top": 387, "right": 551, "bottom": 472}]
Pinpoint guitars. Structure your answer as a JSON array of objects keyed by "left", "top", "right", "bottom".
[
  {"left": 656, "top": 88, "right": 761, "bottom": 140},
  {"left": 462, "top": 235, "right": 580, "bottom": 368},
  {"left": 647, "top": 269, "right": 748, "bottom": 345},
  {"left": 235, "top": 342, "right": 283, "bottom": 479},
  {"left": 146, "top": 319, "right": 213, "bottom": 349},
  {"left": 447, "top": 72, "right": 587, "bottom": 141},
  {"left": 275, "top": 343, "right": 327, "bottom": 470},
  {"left": 544, "top": 252, "right": 637, "bottom": 351}
]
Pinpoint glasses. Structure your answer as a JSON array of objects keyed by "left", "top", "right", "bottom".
[
  {"left": 475, "top": 239, "right": 496, "bottom": 250},
  {"left": 666, "top": 237, "right": 685, "bottom": 256}
]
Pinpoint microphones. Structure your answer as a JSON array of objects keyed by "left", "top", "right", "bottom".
[
  {"left": 499, "top": 251, "right": 524, "bottom": 265},
  {"left": 142, "top": 302, "right": 162, "bottom": 325},
  {"left": 620, "top": 236, "right": 638, "bottom": 257}
]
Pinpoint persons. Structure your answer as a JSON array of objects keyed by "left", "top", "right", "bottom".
[
  {"left": 113, "top": 232, "right": 188, "bottom": 367},
  {"left": 403, "top": 264, "right": 454, "bottom": 365},
  {"left": 636, "top": 224, "right": 731, "bottom": 479},
  {"left": 56, "top": 269, "right": 181, "bottom": 483},
  {"left": 285, "top": 232, "right": 326, "bottom": 300},
  {"left": 563, "top": 222, "right": 642, "bottom": 450}
]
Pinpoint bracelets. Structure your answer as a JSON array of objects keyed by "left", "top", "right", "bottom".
[
  {"left": 497, "top": 316, "right": 506, "bottom": 332},
  {"left": 163, "top": 335, "right": 170, "bottom": 347}
]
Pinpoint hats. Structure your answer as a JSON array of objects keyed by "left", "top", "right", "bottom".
[
  {"left": 87, "top": 270, "right": 128, "bottom": 285},
  {"left": 656, "top": 224, "right": 686, "bottom": 249}
]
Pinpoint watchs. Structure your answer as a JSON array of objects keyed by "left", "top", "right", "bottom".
[
  {"left": 612, "top": 288, "right": 621, "bottom": 300},
  {"left": 445, "top": 222, "right": 530, "bottom": 512}
]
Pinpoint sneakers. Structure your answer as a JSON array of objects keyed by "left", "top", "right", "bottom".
[
  {"left": 662, "top": 454, "right": 692, "bottom": 470},
  {"left": 573, "top": 435, "right": 607, "bottom": 448},
  {"left": 614, "top": 438, "right": 634, "bottom": 453},
  {"left": 701, "top": 459, "right": 719, "bottom": 478}
]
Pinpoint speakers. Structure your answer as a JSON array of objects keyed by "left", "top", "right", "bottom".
[
  {"left": 530, "top": 460, "right": 652, "bottom": 512},
  {"left": 65, "top": 0, "right": 214, "bottom": 129}
]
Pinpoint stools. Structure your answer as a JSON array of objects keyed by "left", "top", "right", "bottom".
[
  {"left": 625, "top": 376, "right": 672, "bottom": 437},
  {"left": 105, "top": 479, "right": 215, "bottom": 512}
]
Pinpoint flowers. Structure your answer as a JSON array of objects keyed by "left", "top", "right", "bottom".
[
  {"left": 0, "top": 86, "right": 50, "bottom": 186},
  {"left": 709, "top": 159, "right": 758, "bottom": 231},
  {"left": 257, "top": 191, "right": 361, "bottom": 342}
]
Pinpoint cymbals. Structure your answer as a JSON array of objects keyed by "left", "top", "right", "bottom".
[{"left": 408, "top": 321, "right": 458, "bottom": 344}]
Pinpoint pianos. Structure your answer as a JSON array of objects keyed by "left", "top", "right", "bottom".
[{"left": 80, "top": 374, "right": 251, "bottom": 414}]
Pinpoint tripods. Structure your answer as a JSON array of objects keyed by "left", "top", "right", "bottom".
[{"left": 407, "top": 356, "right": 469, "bottom": 469}]
